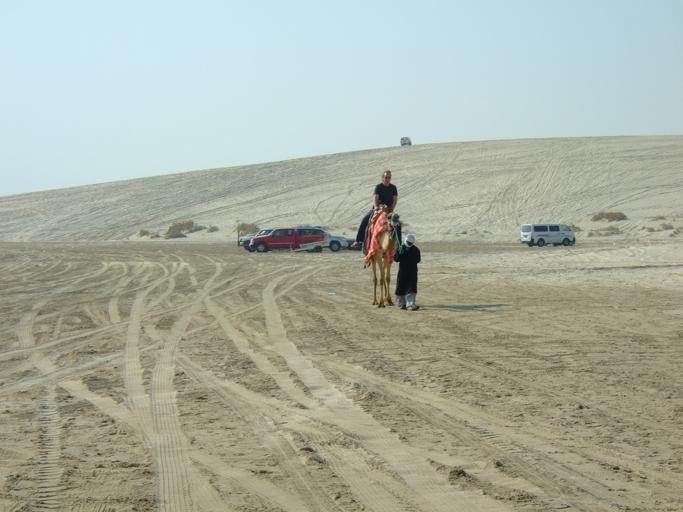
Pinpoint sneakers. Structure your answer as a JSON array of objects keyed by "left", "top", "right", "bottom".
[
  {"left": 407, "top": 306, "right": 415, "bottom": 311},
  {"left": 400, "top": 305, "right": 405, "bottom": 309},
  {"left": 350, "top": 242, "right": 362, "bottom": 248}
]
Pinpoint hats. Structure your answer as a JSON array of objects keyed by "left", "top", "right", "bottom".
[{"left": 405, "top": 235, "right": 415, "bottom": 243}]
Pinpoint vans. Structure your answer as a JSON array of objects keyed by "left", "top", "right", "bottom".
[{"left": 520, "top": 223, "right": 576, "bottom": 248}]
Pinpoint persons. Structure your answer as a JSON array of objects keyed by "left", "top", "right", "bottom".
[
  {"left": 349, "top": 170, "right": 401, "bottom": 250},
  {"left": 393, "top": 232, "right": 420, "bottom": 310}
]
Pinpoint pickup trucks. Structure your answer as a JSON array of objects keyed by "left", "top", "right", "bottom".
[{"left": 238, "top": 225, "right": 349, "bottom": 253}]
